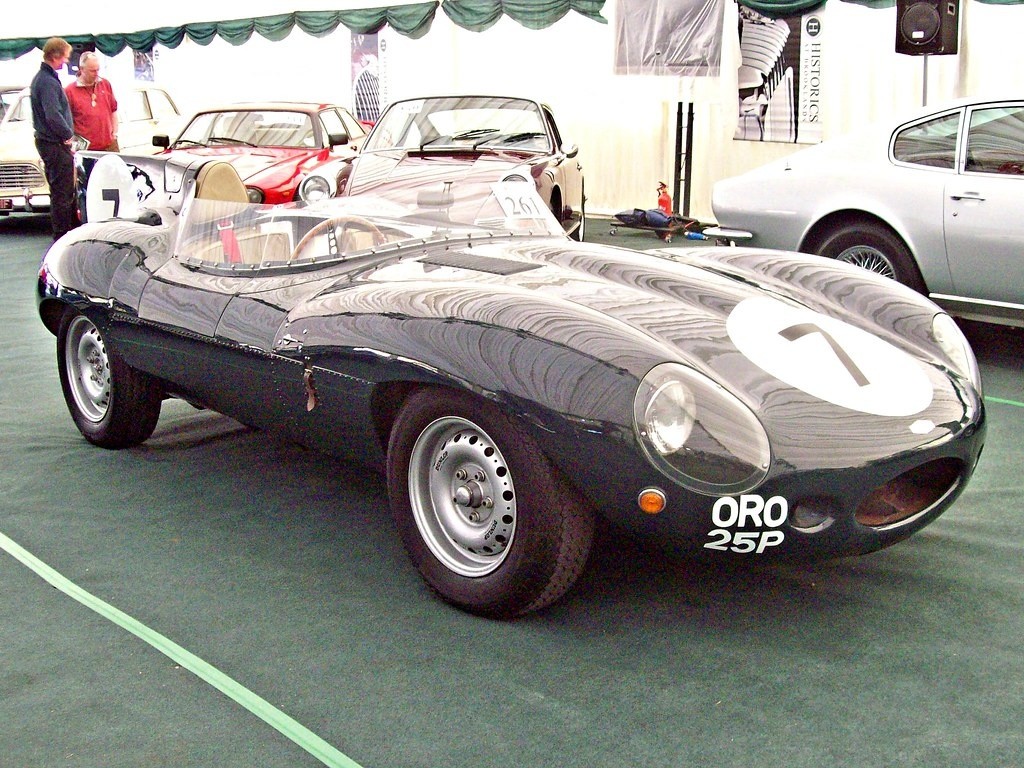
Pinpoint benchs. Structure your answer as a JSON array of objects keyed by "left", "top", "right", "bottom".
[{"left": 253, "top": 127, "right": 330, "bottom": 147}]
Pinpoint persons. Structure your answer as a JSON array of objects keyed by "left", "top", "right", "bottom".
[
  {"left": 61, "top": 51, "right": 120, "bottom": 226},
  {"left": 30, "top": 37, "right": 82, "bottom": 242}
]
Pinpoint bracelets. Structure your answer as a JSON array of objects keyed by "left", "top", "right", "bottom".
[{"left": 113, "top": 133, "right": 119, "bottom": 136}]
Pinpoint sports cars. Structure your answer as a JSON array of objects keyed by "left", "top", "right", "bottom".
[{"left": 33, "top": 159, "right": 987, "bottom": 620}]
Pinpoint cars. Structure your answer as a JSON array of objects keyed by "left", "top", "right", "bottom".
[
  {"left": 151, "top": 102, "right": 378, "bottom": 215},
  {"left": 0, "top": 80, "right": 182, "bottom": 217},
  {"left": 703, "top": 93, "right": 1024, "bottom": 331},
  {"left": 343, "top": 94, "right": 586, "bottom": 240}
]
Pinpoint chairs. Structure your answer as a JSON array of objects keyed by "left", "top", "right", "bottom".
[
  {"left": 223, "top": 117, "right": 258, "bottom": 146},
  {"left": 174, "top": 159, "right": 293, "bottom": 263},
  {"left": 293, "top": 114, "right": 329, "bottom": 147}
]
